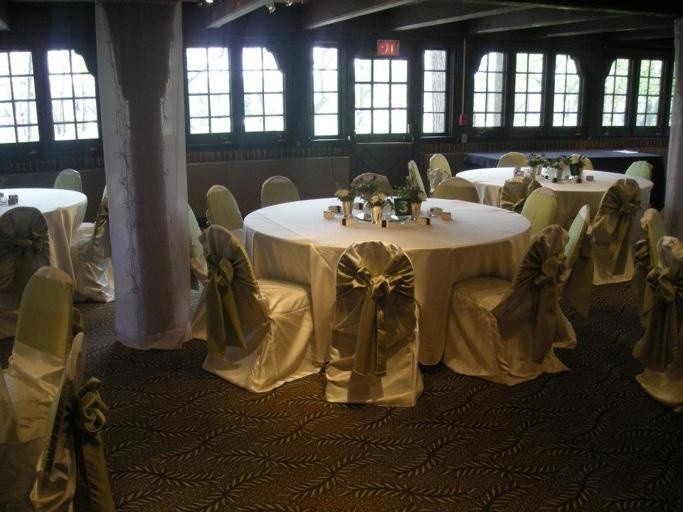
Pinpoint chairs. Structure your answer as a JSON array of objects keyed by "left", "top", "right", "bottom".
[{"left": 0, "top": 265, "right": 85, "bottom": 511}]
